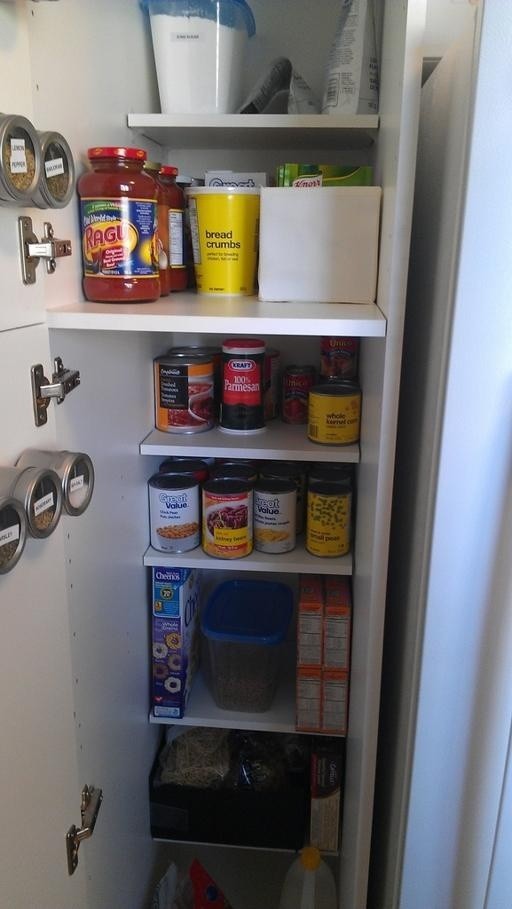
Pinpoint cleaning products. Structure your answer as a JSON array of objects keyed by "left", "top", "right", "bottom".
[
  {"left": 321, "top": 1, "right": 377, "bottom": 118},
  {"left": 238, "top": 55, "right": 322, "bottom": 116}
]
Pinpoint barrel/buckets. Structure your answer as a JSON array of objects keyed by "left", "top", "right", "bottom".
[{"left": 279, "top": 844, "right": 336, "bottom": 909}]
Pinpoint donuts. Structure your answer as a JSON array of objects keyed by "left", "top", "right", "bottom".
[{"left": 153, "top": 632, "right": 181, "bottom": 694}]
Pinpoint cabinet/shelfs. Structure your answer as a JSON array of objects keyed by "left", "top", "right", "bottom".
[{"left": 0, "top": 0, "right": 430, "bottom": 909}]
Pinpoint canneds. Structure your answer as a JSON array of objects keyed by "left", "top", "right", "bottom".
[
  {"left": 152, "top": 348, "right": 223, "bottom": 435},
  {"left": 77, "top": 149, "right": 203, "bottom": 303},
  {"left": 148, "top": 456, "right": 354, "bottom": 558},
  {"left": 281, "top": 338, "right": 361, "bottom": 447}
]
